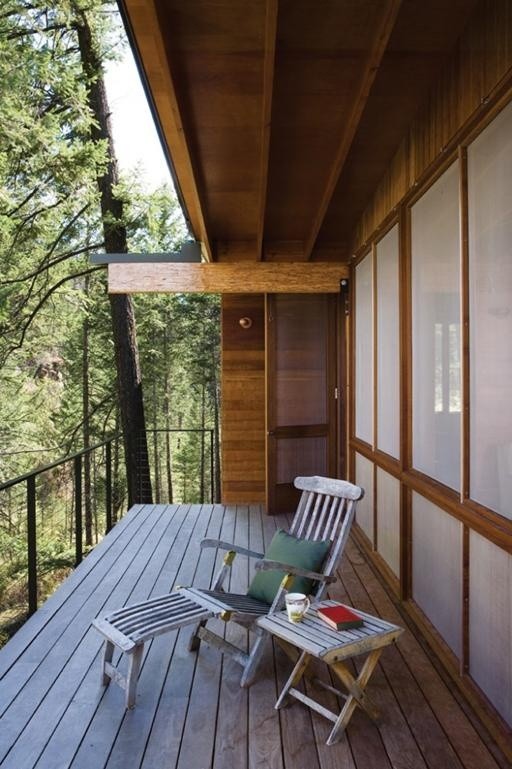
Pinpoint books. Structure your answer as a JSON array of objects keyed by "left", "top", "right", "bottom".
[{"left": 317, "top": 605, "right": 363, "bottom": 631}]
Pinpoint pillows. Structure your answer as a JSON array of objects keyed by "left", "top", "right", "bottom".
[{"left": 246, "top": 527, "right": 332, "bottom": 609}]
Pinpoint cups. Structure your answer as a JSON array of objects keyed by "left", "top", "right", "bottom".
[{"left": 285, "top": 592, "right": 310, "bottom": 624}]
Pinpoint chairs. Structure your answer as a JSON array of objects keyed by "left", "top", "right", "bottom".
[{"left": 91, "top": 476, "right": 365, "bottom": 711}]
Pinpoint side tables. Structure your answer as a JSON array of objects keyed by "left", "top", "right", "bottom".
[{"left": 254, "top": 599, "right": 405, "bottom": 746}]
